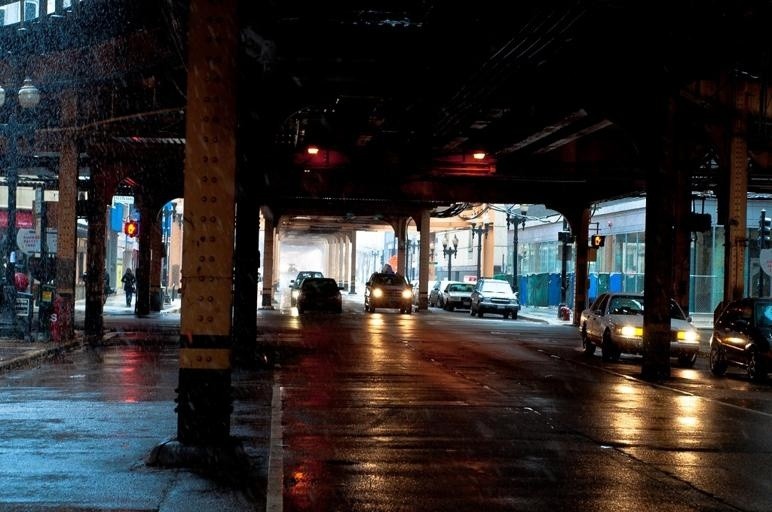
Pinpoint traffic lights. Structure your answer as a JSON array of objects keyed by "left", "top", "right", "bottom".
[
  {"left": 760, "top": 217, "right": 772, "bottom": 249},
  {"left": 591, "top": 236, "right": 606, "bottom": 249},
  {"left": 125, "top": 223, "right": 138, "bottom": 237}
]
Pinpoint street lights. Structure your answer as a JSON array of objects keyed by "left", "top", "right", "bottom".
[
  {"left": 471, "top": 214, "right": 489, "bottom": 279},
  {"left": 162, "top": 200, "right": 183, "bottom": 287},
  {"left": 0, "top": 75, "right": 39, "bottom": 336},
  {"left": 441, "top": 234, "right": 459, "bottom": 280},
  {"left": 503, "top": 204, "right": 528, "bottom": 294},
  {"left": 409, "top": 237, "right": 421, "bottom": 280}
]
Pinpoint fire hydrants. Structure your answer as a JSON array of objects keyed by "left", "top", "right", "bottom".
[{"left": 561, "top": 306, "right": 571, "bottom": 322}]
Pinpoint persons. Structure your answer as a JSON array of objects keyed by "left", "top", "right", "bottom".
[{"left": 121, "top": 268, "right": 136, "bottom": 308}]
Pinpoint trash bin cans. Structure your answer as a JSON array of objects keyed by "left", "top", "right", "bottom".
[{"left": 49, "top": 289, "right": 73, "bottom": 342}]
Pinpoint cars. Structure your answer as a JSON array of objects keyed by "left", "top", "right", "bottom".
[
  {"left": 364, "top": 272, "right": 520, "bottom": 320},
  {"left": 288, "top": 271, "right": 344, "bottom": 313},
  {"left": 580, "top": 292, "right": 772, "bottom": 385}
]
